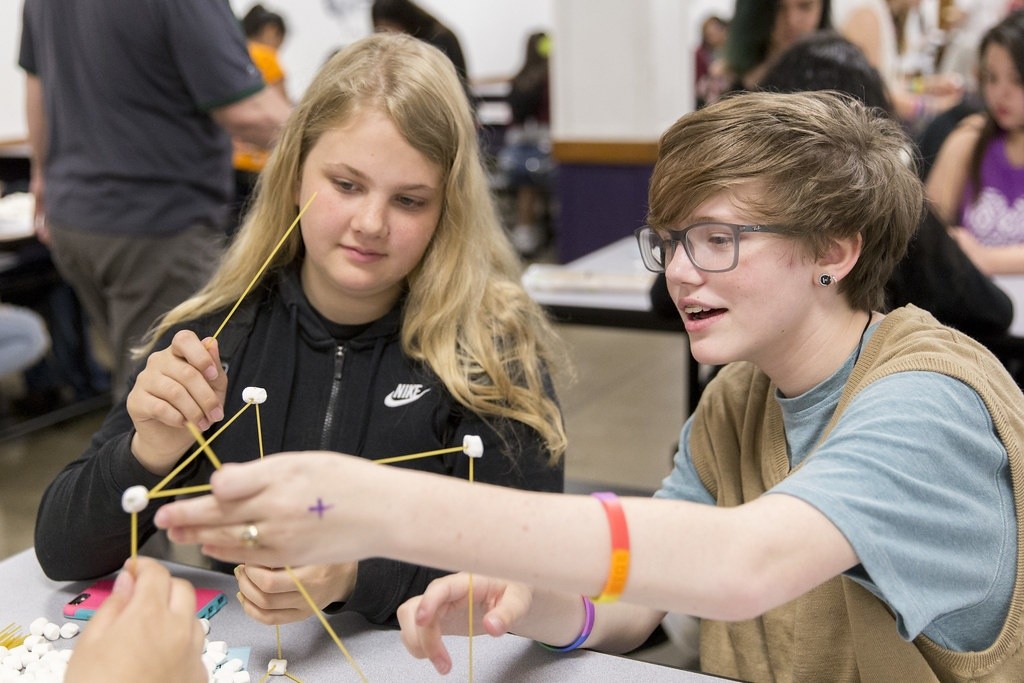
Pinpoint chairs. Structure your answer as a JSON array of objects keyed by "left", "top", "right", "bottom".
[{"left": 470, "top": 33, "right": 557, "bottom": 257}]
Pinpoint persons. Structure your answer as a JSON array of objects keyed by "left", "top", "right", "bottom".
[
  {"left": 19, "top": 0, "right": 549, "bottom": 403},
  {"left": 153, "top": 90, "right": 1022, "bottom": 683},
  {"left": 649, "top": 0, "right": 1024, "bottom": 355},
  {"left": 64, "top": 556, "right": 207, "bottom": 683},
  {"left": 35, "top": 31, "right": 566, "bottom": 628}
]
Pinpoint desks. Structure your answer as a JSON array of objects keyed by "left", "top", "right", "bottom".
[
  {"left": 0, "top": 545, "right": 749, "bottom": 683},
  {"left": 521, "top": 229, "right": 1024, "bottom": 498}
]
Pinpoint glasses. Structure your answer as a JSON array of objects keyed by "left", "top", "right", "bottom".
[{"left": 632, "top": 220, "right": 808, "bottom": 275}]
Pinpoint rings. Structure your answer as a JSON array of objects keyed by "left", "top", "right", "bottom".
[{"left": 240, "top": 523, "right": 259, "bottom": 550}]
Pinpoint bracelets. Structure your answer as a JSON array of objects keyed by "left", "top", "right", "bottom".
[
  {"left": 586, "top": 490, "right": 631, "bottom": 604},
  {"left": 535, "top": 594, "right": 595, "bottom": 653}
]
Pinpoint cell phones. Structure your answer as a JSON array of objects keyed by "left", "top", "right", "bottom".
[{"left": 64, "top": 580, "right": 228, "bottom": 620}]
